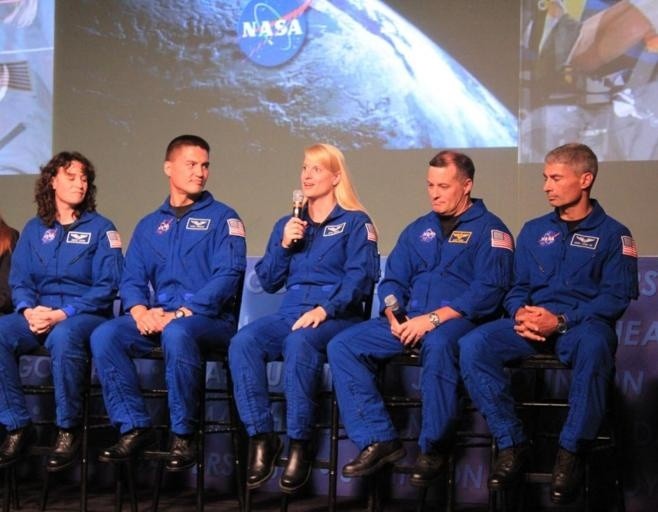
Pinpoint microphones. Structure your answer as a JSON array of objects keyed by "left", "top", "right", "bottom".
[
  {"left": 384, "top": 295, "right": 407, "bottom": 325},
  {"left": 292, "top": 190, "right": 305, "bottom": 242}
]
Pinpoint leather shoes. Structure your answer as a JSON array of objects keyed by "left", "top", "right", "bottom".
[
  {"left": 246, "top": 431, "right": 283, "bottom": 490},
  {"left": 411, "top": 453, "right": 450, "bottom": 486},
  {"left": 99, "top": 428, "right": 150, "bottom": 462},
  {"left": 44, "top": 430, "right": 84, "bottom": 471},
  {"left": 163, "top": 433, "right": 199, "bottom": 472},
  {"left": 280, "top": 439, "right": 317, "bottom": 493},
  {"left": 551, "top": 448, "right": 581, "bottom": 503},
  {"left": 487, "top": 439, "right": 533, "bottom": 488},
  {"left": 0, "top": 426, "right": 37, "bottom": 467},
  {"left": 343, "top": 440, "right": 406, "bottom": 477}
]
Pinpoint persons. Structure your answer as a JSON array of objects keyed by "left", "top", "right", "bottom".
[
  {"left": 0, "top": 216, "right": 19, "bottom": 316},
  {"left": 327, "top": 150, "right": 515, "bottom": 485},
  {"left": 457, "top": 143, "right": 639, "bottom": 505},
  {"left": 228, "top": 144, "right": 381, "bottom": 493},
  {"left": 90, "top": 135, "right": 248, "bottom": 473},
  {"left": 0, "top": 152, "right": 123, "bottom": 471}
]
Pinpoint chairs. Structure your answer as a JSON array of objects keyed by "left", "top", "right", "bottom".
[{"left": 0, "top": 251, "right": 624, "bottom": 512}]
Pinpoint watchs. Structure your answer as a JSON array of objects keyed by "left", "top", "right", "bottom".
[
  {"left": 430, "top": 311, "right": 440, "bottom": 328},
  {"left": 556, "top": 315, "right": 568, "bottom": 334},
  {"left": 174, "top": 309, "right": 185, "bottom": 319}
]
